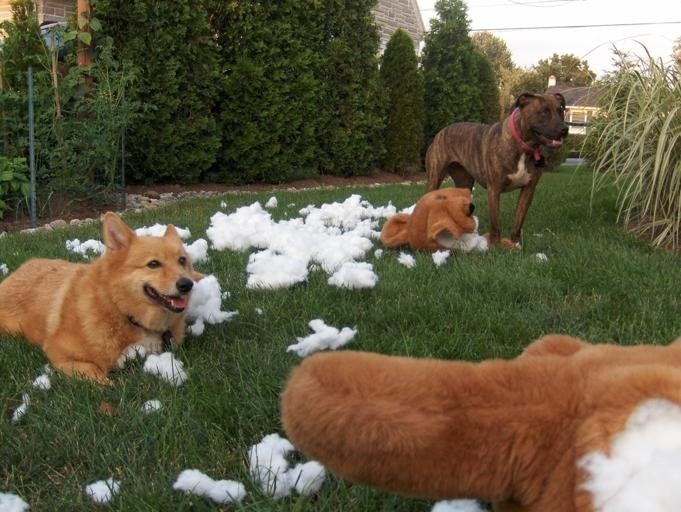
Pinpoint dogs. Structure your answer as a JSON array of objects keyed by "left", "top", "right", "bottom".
[
  {"left": 424, "top": 91, "right": 570, "bottom": 249},
  {"left": 0, "top": 210, "right": 206, "bottom": 390}
]
